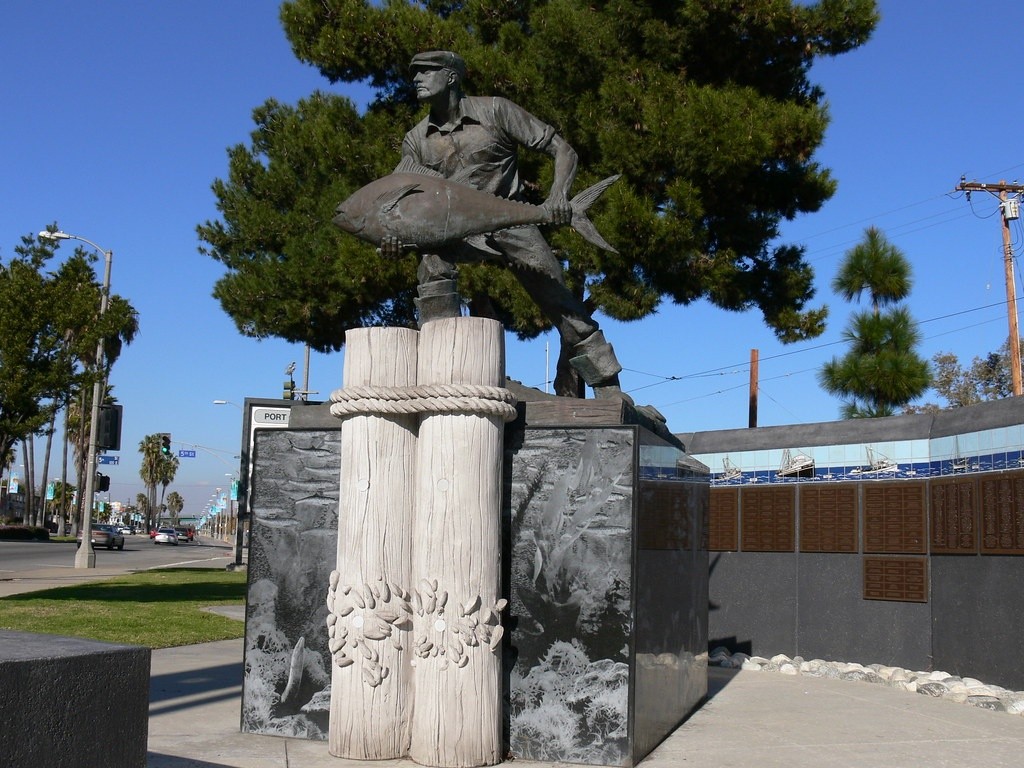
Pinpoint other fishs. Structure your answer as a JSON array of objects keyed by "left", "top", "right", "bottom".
[{"left": 332, "top": 155, "right": 621, "bottom": 256}]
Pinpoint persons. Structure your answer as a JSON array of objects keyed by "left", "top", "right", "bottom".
[{"left": 378, "top": 50, "right": 667, "bottom": 424}]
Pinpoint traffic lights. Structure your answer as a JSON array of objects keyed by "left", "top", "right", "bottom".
[
  {"left": 95, "top": 475, "right": 111, "bottom": 493},
  {"left": 282, "top": 380, "right": 296, "bottom": 400},
  {"left": 159, "top": 432, "right": 173, "bottom": 456}
]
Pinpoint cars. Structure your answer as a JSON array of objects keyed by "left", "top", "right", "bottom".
[
  {"left": 149, "top": 527, "right": 159, "bottom": 539},
  {"left": 187, "top": 528, "right": 194, "bottom": 541},
  {"left": 76, "top": 522, "right": 125, "bottom": 550},
  {"left": 115, "top": 524, "right": 144, "bottom": 535},
  {"left": 175, "top": 528, "right": 192, "bottom": 543},
  {"left": 154, "top": 527, "right": 179, "bottom": 545}
]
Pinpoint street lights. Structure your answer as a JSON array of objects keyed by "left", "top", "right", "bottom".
[
  {"left": 3, "top": 464, "right": 25, "bottom": 518},
  {"left": 36, "top": 230, "right": 114, "bottom": 570},
  {"left": 42, "top": 477, "right": 61, "bottom": 528}
]
